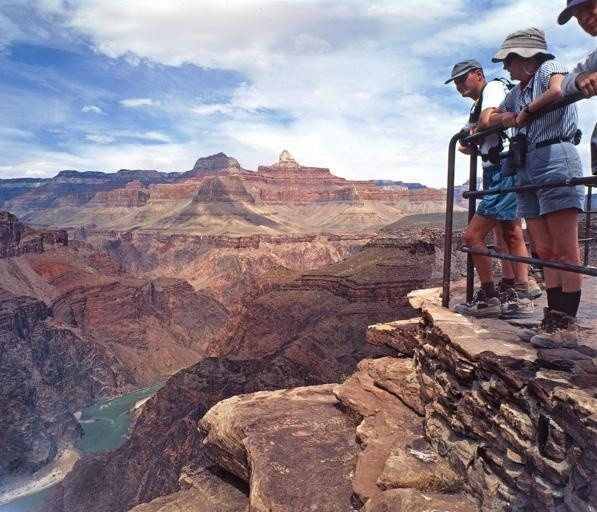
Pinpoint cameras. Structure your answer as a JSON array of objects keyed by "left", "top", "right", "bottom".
[{"left": 509, "top": 133, "right": 528, "bottom": 168}]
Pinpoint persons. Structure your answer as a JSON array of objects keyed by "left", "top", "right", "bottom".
[
  {"left": 488, "top": 27, "right": 584, "bottom": 348},
  {"left": 557, "top": 1, "right": 597, "bottom": 177},
  {"left": 458, "top": 142, "right": 542, "bottom": 299},
  {"left": 445, "top": 60, "right": 534, "bottom": 318}
]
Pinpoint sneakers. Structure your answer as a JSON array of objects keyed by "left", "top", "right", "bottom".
[
  {"left": 454, "top": 275, "right": 542, "bottom": 317},
  {"left": 530, "top": 310, "right": 579, "bottom": 348},
  {"left": 517, "top": 306, "right": 564, "bottom": 342}
]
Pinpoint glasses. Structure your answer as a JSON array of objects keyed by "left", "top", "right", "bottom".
[{"left": 502, "top": 55, "right": 516, "bottom": 67}]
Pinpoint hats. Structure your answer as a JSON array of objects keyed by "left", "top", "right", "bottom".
[
  {"left": 558, "top": 1, "right": 595, "bottom": 26},
  {"left": 444, "top": 58, "right": 482, "bottom": 85},
  {"left": 492, "top": 24, "right": 556, "bottom": 64}
]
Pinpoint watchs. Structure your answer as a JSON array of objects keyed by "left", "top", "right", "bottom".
[{"left": 521, "top": 100, "right": 532, "bottom": 113}]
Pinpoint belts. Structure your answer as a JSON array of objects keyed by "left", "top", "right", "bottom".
[{"left": 535, "top": 137, "right": 569, "bottom": 149}]
